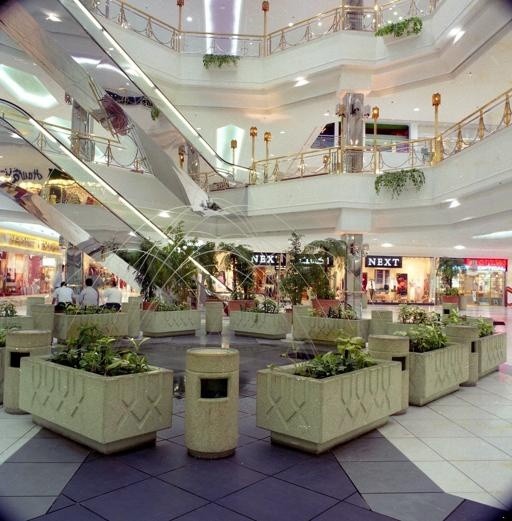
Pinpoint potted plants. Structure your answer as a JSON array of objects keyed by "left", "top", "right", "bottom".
[
  {"left": 0, "top": 219, "right": 512, "bottom": 453},
  {"left": 374, "top": 168, "right": 426, "bottom": 201},
  {"left": 202, "top": 53, "right": 241, "bottom": 71},
  {"left": 373, "top": 16, "right": 422, "bottom": 47}
]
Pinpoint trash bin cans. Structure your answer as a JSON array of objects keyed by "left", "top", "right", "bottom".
[
  {"left": 122, "top": 301, "right": 143, "bottom": 340},
  {"left": 25, "top": 295, "right": 46, "bottom": 315},
  {"left": 368, "top": 308, "right": 395, "bottom": 334},
  {"left": 204, "top": 300, "right": 225, "bottom": 337},
  {"left": 459, "top": 295, "right": 467, "bottom": 310},
  {"left": 366, "top": 333, "right": 413, "bottom": 417},
  {"left": 183, "top": 346, "right": 241, "bottom": 463},
  {"left": 440, "top": 301, "right": 458, "bottom": 324},
  {"left": 128, "top": 295, "right": 144, "bottom": 316},
  {"left": 25, "top": 301, "right": 57, "bottom": 331},
  {"left": 2, "top": 327, "right": 53, "bottom": 418},
  {"left": 449, "top": 320, "right": 482, "bottom": 387},
  {"left": 291, "top": 303, "right": 313, "bottom": 343}
]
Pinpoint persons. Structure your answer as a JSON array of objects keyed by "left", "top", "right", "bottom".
[
  {"left": 54, "top": 265, "right": 64, "bottom": 287},
  {"left": 78, "top": 278, "right": 99, "bottom": 310},
  {"left": 100, "top": 281, "right": 122, "bottom": 311},
  {"left": 52, "top": 282, "right": 76, "bottom": 312}
]
